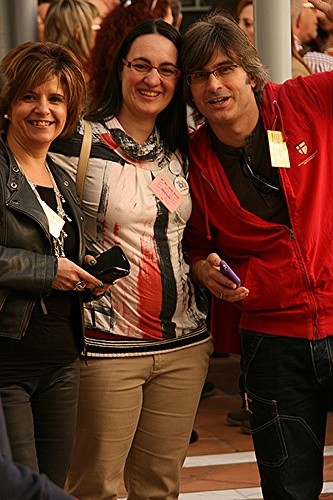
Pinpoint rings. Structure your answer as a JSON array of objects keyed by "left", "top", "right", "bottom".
[
  {"left": 73, "top": 280, "right": 87, "bottom": 292},
  {"left": 220, "top": 292, "right": 223, "bottom": 299}
]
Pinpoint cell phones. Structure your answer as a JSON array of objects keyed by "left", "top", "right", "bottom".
[
  {"left": 219, "top": 260, "right": 240, "bottom": 289},
  {"left": 94, "top": 267, "right": 130, "bottom": 284}
]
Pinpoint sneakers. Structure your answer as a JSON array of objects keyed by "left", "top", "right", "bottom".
[
  {"left": 200, "top": 381, "right": 216, "bottom": 397},
  {"left": 226, "top": 411, "right": 251, "bottom": 434}
]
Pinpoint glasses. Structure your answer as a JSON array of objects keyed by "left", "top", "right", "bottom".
[
  {"left": 121, "top": 58, "right": 181, "bottom": 80},
  {"left": 183, "top": 64, "right": 241, "bottom": 87}
]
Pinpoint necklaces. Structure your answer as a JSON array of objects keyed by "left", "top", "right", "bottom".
[{"left": 12, "top": 153, "right": 72, "bottom": 259}]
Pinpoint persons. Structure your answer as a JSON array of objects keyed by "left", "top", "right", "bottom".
[
  {"left": 0, "top": 0, "right": 333, "bottom": 444},
  {"left": 182, "top": 15, "right": 333, "bottom": 500},
  {"left": 47, "top": 19, "right": 214, "bottom": 500},
  {"left": 0, "top": 42, "right": 123, "bottom": 492}
]
